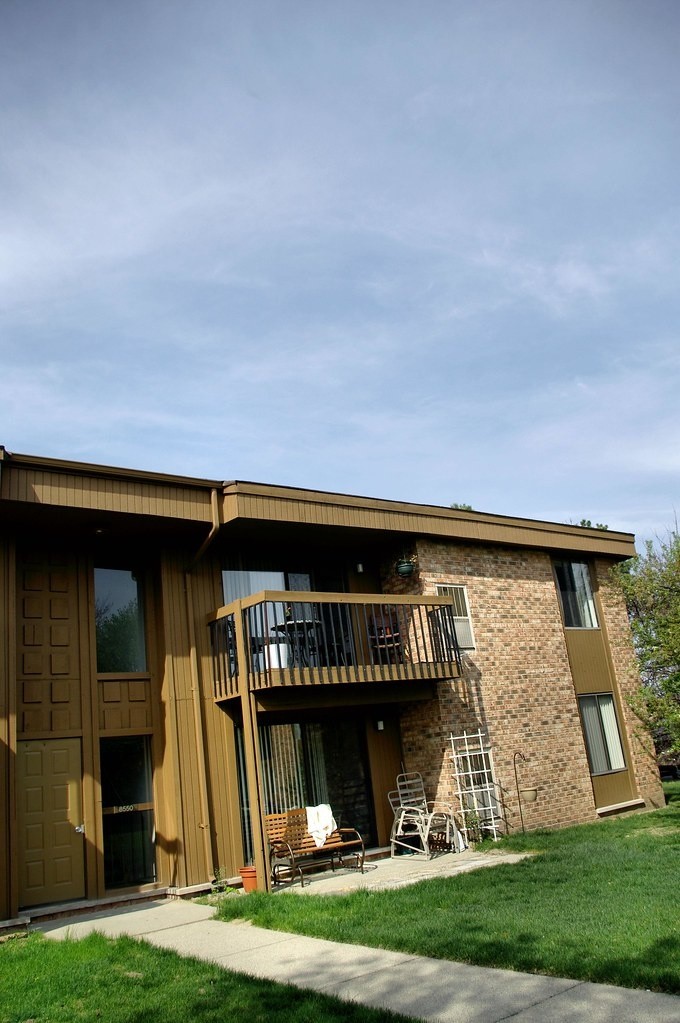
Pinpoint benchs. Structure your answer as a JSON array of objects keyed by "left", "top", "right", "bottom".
[{"left": 261, "top": 801, "right": 367, "bottom": 886}]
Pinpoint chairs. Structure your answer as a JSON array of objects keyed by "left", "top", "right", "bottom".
[
  {"left": 387, "top": 772, "right": 461, "bottom": 861},
  {"left": 371, "top": 611, "right": 401, "bottom": 663},
  {"left": 228, "top": 623, "right": 264, "bottom": 675}
]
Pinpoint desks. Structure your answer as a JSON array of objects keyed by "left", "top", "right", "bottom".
[{"left": 271, "top": 619, "right": 323, "bottom": 667}]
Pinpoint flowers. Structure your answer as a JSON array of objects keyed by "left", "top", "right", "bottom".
[
  {"left": 281, "top": 606, "right": 292, "bottom": 615},
  {"left": 393, "top": 550, "right": 418, "bottom": 565}
]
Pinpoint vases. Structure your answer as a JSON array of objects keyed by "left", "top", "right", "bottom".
[
  {"left": 520, "top": 788, "right": 538, "bottom": 802},
  {"left": 238, "top": 867, "right": 258, "bottom": 893},
  {"left": 397, "top": 563, "right": 414, "bottom": 577}
]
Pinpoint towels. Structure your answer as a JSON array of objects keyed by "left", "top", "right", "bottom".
[{"left": 305, "top": 803, "right": 338, "bottom": 848}]
